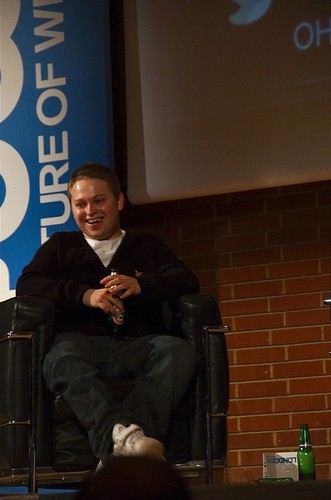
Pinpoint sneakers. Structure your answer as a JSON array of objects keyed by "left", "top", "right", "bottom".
[{"left": 112, "top": 424, "right": 164, "bottom": 457}]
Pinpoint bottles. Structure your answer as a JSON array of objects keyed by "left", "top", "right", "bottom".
[
  {"left": 297, "top": 423, "right": 316, "bottom": 480},
  {"left": 106, "top": 267, "right": 125, "bottom": 338}
]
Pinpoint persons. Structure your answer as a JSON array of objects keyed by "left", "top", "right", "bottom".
[{"left": 15, "top": 162, "right": 199, "bottom": 478}]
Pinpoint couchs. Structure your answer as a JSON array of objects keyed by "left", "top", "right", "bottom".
[{"left": 0, "top": 291, "right": 231, "bottom": 495}]
